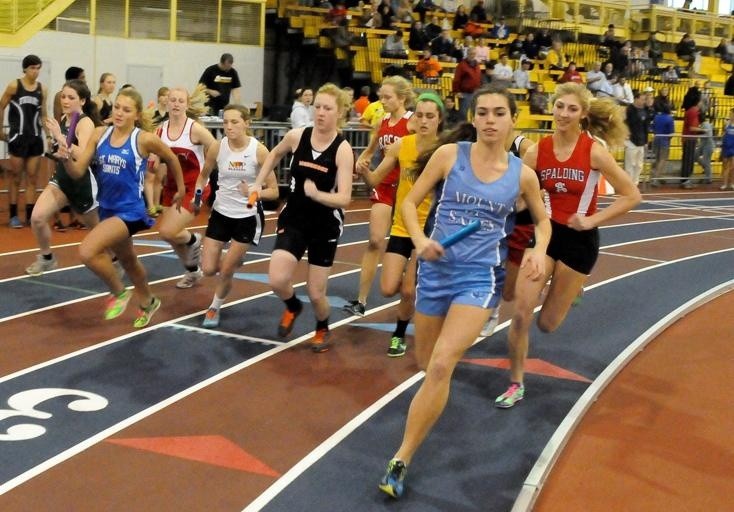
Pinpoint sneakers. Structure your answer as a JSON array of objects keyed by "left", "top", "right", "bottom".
[
  {"left": 105, "top": 233, "right": 229, "bottom": 328},
  {"left": 148, "top": 205, "right": 164, "bottom": 217},
  {"left": 387, "top": 336, "right": 407, "bottom": 357},
  {"left": 311, "top": 329, "right": 331, "bottom": 353},
  {"left": 278, "top": 303, "right": 303, "bottom": 337},
  {"left": 25, "top": 256, "right": 57, "bottom": 274},
  {"left": 343, "top": 300, "right": 365, "bottom": 317},
  {"left": 652, "top": 178, "right": 734, "bottom": 190},
  {"left": 480, "top": 317, "right": 498, "bottom": 337},
  {"left": 379, "top": 459, "right": 407, "bottom": 498},
  {"left": 10, "top": 216, "right": 24, "bottom": 228},
  {"left": 572, "top": 277, "right": 584, "bottom": 307},
  {"left": 494, "top": 383, "right": 526, "bottom": 408},
  {"left": 53, "top": 220, "right": 86, "bottom": 231}
]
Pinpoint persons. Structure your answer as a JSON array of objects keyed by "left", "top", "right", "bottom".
[
  {"left": 246, "top": 83, "right": 357, "bottom": 355},
  {"left": 0, "top": 54, "right": 387, "bottom": 323},
  {"left": 320, "top": 1, "right": 734, "bottom": 191},
  {"left": 353, "top": 91, "right": 445, "bottom": 357},
  {"left": 372, "top": 84, "right": 555, "bottom": 501},
  {"left": 491, "top": 82, "right": 646, "bottom": 410}
]
